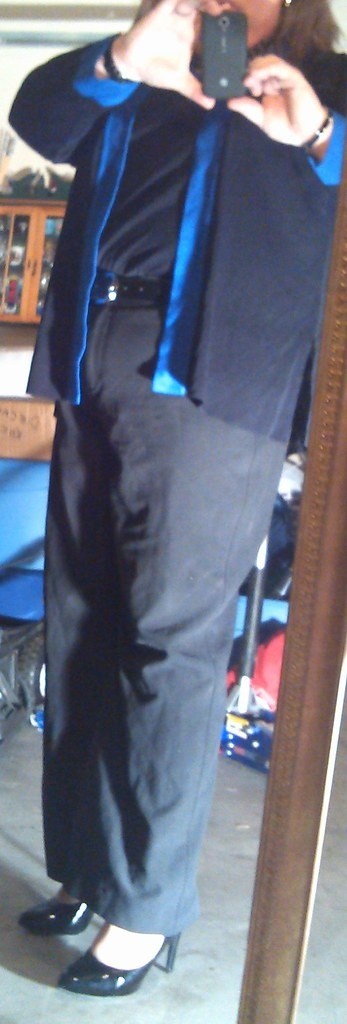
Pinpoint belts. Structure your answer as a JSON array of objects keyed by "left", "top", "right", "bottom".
[{"left": 93, "top": 272, "right": 171, "bottom": 304}]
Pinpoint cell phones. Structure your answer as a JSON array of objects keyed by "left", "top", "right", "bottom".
[{"left": 200, "top": 11, "right": 247, "bottom": 98}]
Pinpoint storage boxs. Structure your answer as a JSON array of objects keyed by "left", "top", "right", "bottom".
[{"left": 0, "top": 395, "right": 59, "bottom": 461}]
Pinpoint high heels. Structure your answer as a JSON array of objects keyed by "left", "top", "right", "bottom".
[
  {"left": 58, "top": 936, "right": 181, "bottom": 997},
  {"left": 18, "top": 886, "right": 94, "bottom": 935}
]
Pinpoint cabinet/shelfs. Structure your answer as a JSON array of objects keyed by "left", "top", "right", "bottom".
[{"left": 0, "top": 196, "right": 68, "bottom": 322}]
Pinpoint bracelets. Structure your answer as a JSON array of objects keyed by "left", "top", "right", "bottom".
[
  {"left": 99, "top": 44, "right": 125, "bottom": 86},
  {"left": 303, "top": 110, "right": 336, "bottom": 150}
]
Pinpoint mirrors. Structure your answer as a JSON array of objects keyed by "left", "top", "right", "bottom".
[{"left": 0, "top": 0, "right": 347, "bottom": 1023}]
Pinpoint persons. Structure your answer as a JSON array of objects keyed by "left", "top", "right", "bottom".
[{"left": 2, "top": 0, "right": 347, "bottom": 1005}]
